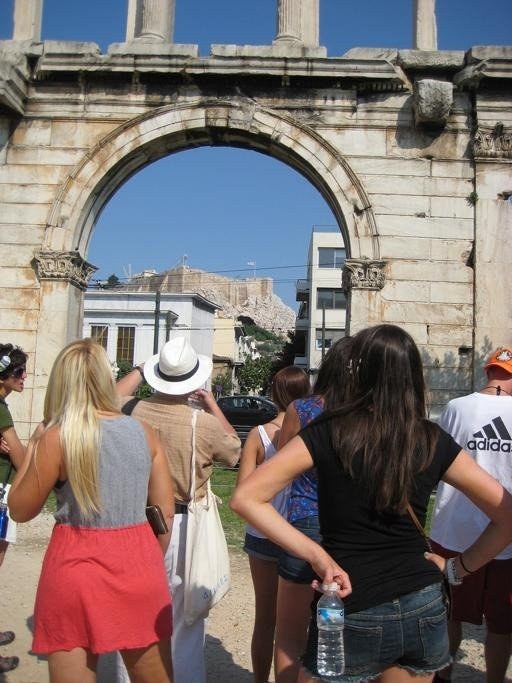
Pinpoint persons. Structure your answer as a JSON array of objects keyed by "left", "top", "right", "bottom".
[
  {"left": 230, "top": 324, "right": 512, "bottom": 683},
  {"left": 231, "top": 365, "right": 313, "bottom": 683},
  {"left": 112, "top": 337, "right": 241, "bottom": 683},
  {"left": 274, "top": 335, "right": 353, "bottom": 683},
  {"left": 0, "top": 343, "right": 26, "bottom": 673},
  {"left": 7, "top": 338, "right": 177, "bottom": 682},
  {"left": 429, "top": 344, "right": 512, "bottom": 683}
]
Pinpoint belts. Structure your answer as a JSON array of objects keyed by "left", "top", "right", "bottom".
[{"left": 175, "top": 504, "right": 188, "bottom": 514}]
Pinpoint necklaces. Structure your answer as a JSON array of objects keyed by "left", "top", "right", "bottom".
[{"left": 482, "top": 386, "right": 510, "bottom": 396}]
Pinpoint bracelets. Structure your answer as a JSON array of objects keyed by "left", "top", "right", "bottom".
[
  {"left": 135, "top": 366, "right": 144, "bottom": 376},
  {"left": 460, "top": 555, "right": 473, "bottom": 574},
  {"left": 447, "top": 558, "right": 464, "bottom": 585}
]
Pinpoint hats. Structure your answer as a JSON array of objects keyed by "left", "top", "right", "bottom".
[
  {"left": 485, "top": 347, "right": 512, "bottom": 373},
  {"left": 143, "top": 337, "right": 213, "bottom": 395}
]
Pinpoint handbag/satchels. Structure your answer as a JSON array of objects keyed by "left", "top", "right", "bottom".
[
  {"left": 183, "top": 494, "right": 230, "bottom": 626},
  {"left": 146, "top": 504, "right": 168, "bottom": 534}
]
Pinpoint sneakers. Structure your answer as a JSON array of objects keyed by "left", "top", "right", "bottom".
[
  {"left": 0, "top": 656, "right": 19, "bottom": 671},
  {"left": 0, "top": 631, "right": 15, "bottom": 645}
]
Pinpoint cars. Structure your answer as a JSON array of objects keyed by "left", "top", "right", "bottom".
[{"left": 217, "top": 394, "right": 280, "bottom": 424}]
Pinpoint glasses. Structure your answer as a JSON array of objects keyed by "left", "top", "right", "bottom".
[{"left": 4, "top": 364, "right": 27, "bottom": 377}]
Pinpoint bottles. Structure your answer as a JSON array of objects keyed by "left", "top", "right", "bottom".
[{"left": 316, "top": 580, "right": 344, "bottom": 675}]
